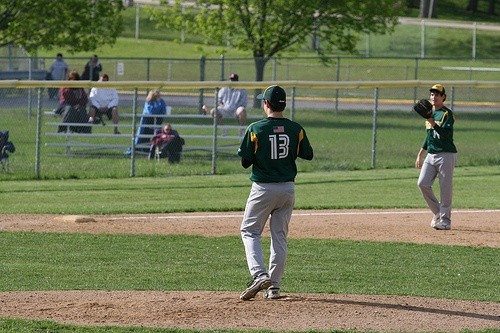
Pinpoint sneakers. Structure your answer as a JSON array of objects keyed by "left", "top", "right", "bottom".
[
  {"left": 433, "top": 218, "right": 451, "bottom": 230},
  {"left": 239, "top": 272, "right": 272, "bottom": 300},
  {"left": 431, "top": 212, "right": 441, "bottom": 228},
  {"left": 263, "top": 286, "right": 280, "bottom": 299}
]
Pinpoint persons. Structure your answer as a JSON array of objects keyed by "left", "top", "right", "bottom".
[
  {"left": 128, "top": 89, "right": 167, "bottom": 151},
  {"left": 54, "top": 72, "right": 91, "bottom": 133},
  {"left": 154, "top": 123, "right": 185, "bottom": 161},
  {"left": 81, "top": 54, "right": 103, "bottom": 96},
  {"left": 415, "top": 83, "right": 458, "bottom": 231},
  {"left": 210, "top": 73, "right": 247, "bottom": 137},
  {"left": 87, "top": 73, "right": 121, "bottom": 135},
  {"left": 44, "top": 52, "right": 69, "bottom": 102},
  {"left": 238, "top": 83, "right": 314, "bottom": 302}
]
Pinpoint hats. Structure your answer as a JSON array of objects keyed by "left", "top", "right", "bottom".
[
  {"left": 257, "top": 85, "right": 286, "bottom": 107},
  {"left": 429, "top": 84, "right": 445, "bottom": 96}
]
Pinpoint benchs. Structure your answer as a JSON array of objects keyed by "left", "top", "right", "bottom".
[{"left": 44, "top": 111, "right": 265, "bottom": 163}]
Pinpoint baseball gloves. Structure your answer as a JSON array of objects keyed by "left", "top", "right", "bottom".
[{"left": 414, "top": 98, "right": 434, "bottom": 119}]
